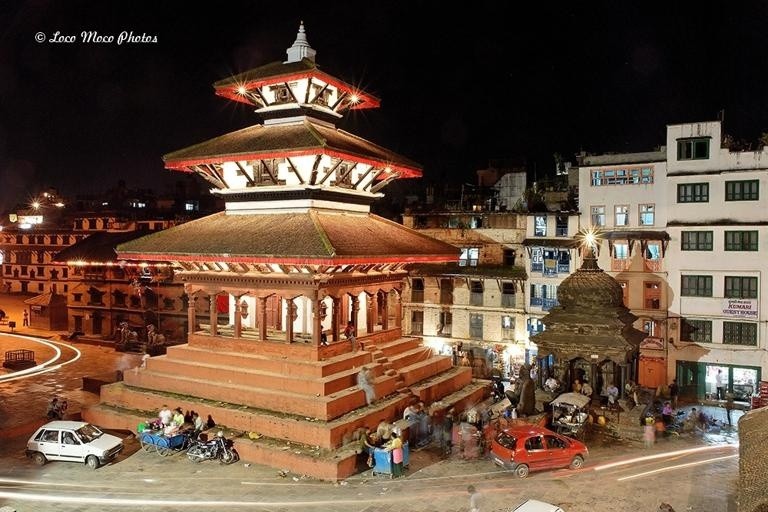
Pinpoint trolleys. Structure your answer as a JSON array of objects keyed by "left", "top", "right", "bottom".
[{"left": 138, "top": 423, "right": 188, "bottom": 456}]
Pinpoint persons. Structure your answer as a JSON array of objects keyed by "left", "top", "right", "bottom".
[
  {"left": 46, "top": 398, "right": 59, "bottom": 416},
  {"left": 715, "top": 370, "right": 724, "bottom": 400},
  {"left": 377, "top": 419, "right": 392, "bottom": 441},
  {"left": 190, "top": 413, "right": 203, "bottom": 443},
  {"left": 158, "top": 405, "right": 171, "bottom": 426},
  {"left": 359, "top": 427, "right": 376, "bottom": 468},
  {"left": 61, "top": 400, "right": 69, "bottom": 413},
  {"left": 320, "top": 326, "right": 328, "bottom": 346},
  {"left": 386, "top": 432, "right": 404, "bottom": 479},
  {"left": 467, "top": 485, "right": 484, "bottom": 512},
  {"left": 22, "top": 308, "right": 28, "bottom": 326},
  {"left": 344, "top": 321, "right": 358, "bottom": 353},
  {"left": 641, "top": 403, "right": 701, "bottom": 447},
  {"left": 724, "top": 392, "right": 735, "bottom": 425},
  {"left": 402, "top": 398, "right": 480, "bottom": 458},
  {"left": 544, "top": 372, "right": 698, "bottom": 411},
  {"left": 172, "top": 407, "right": 185, "bottom": 430},
  {"left": 358, "top": 367, "right": 376, "bottom": 406}
]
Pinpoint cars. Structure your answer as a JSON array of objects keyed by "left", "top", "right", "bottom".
[
  {"left": 25, "top": 420, "right": 126, "bottom": 471},
  {"left": 491, "top": 423, "right": 590, "bottom": 477}
]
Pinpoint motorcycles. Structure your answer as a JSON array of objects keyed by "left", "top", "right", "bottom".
[
  {"left": 490, "top": 375, "right": 505, "bottom": 402},
  {"left": 185, "top": 430, "right": 237, "bottom": 464}
]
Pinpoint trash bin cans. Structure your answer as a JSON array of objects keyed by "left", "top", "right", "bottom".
[{"left": 371, "top": 440, "right": 409, "bottom": 479}]
